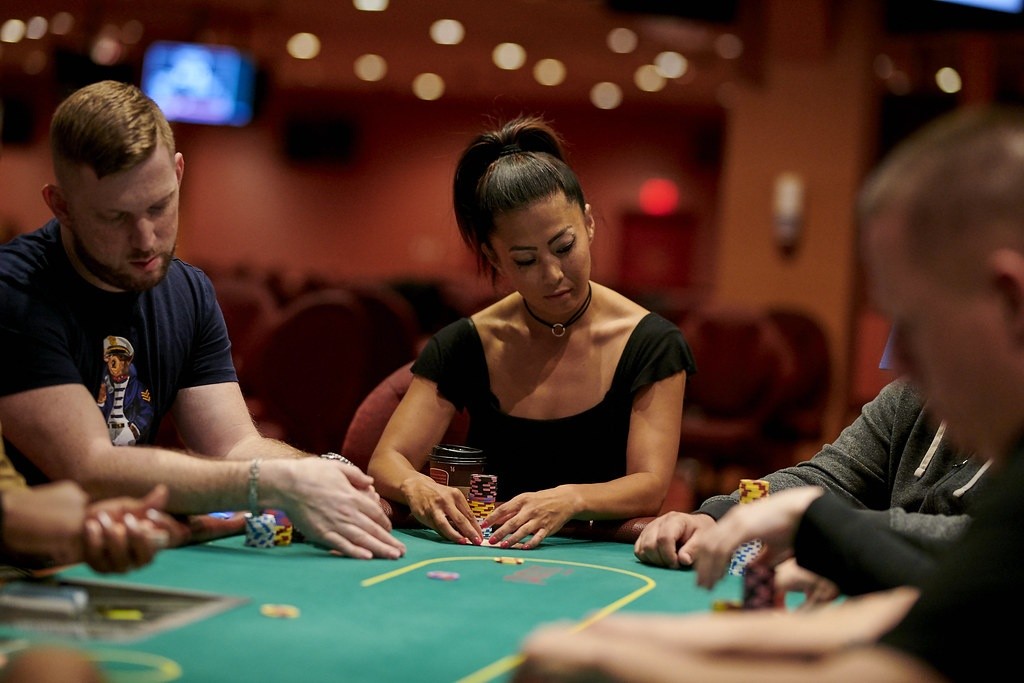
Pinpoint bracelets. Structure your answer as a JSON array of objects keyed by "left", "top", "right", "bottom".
[
  {"left": 247, "top": 458, "right": 265, "bottom": 517},
  {"left": 321, "top": 453, "right": 354, "bottom": 465}
]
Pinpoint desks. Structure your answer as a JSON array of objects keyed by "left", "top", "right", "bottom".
[{"left": 0, "top": 502, "right": 844, "bottom": 683}]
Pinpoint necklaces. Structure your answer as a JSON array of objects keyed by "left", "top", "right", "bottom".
[{"left": 522, "top": 282, "right": 593, "bottom": 337}]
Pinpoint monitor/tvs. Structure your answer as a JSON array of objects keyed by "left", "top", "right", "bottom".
[{"left": 139, "top": 40, "right": 254, "bottom": 129}]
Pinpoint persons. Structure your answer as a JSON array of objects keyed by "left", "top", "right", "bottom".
[
  {"left": 635, "top": 376, "right": 996, "bottom": 612},
  {"left": 367, "top": 117, "right": 697, "bottom": 550},
  {"left": 0, "top": 428, "right": 180, "bottom": 574},
  {"left": 0, "top": 80, "right": 407, "bottom": 559},
  {"left": 520, "top": 107, "right": 1024, "bottom": 683}
]
